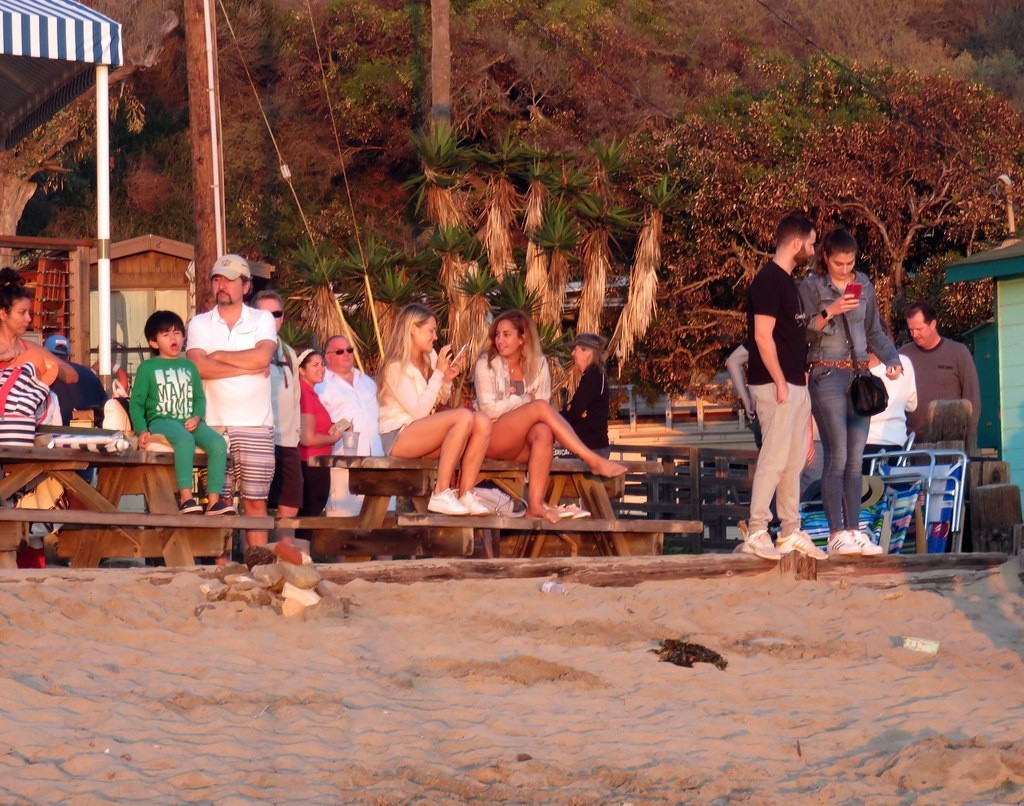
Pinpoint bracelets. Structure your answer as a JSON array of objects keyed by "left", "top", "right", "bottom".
[{"left": 820, "top": 309, "right": 835, "bottom": 329}]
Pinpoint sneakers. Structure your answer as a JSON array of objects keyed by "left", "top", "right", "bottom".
[
  {"left": 427, "top": 487, "right": 489, "bottom": 517},
  {"left": 741, "top": 530, "right": 782, "bottom": 560},
  {"left": 776, "top": 528, "right": 829, "bottom": 560},
  {"left": 827, "top": 531, "right": 883, "bottom": 556},
  {"left": 553, "top": 503, "right": 591, "bottom": 519}
]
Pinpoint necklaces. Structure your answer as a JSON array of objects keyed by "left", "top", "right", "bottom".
[{"left": 508, "top": 366, "right": 520, "bottom": 372}]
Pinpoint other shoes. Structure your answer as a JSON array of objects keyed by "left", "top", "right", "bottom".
[
  {"left": 204, "top": 500, "right": 237, "bottom": 515},
  {"left": 179, "top": 499, "right": 203, "bottom": 514}
]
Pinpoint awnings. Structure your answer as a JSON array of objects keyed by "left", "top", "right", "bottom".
[{"left": 0, "top": 1, "right": 127, "bottom": 393}]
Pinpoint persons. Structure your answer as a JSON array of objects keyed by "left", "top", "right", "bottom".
[
  {"left": 377, "top": 302, "right": 491, "bottom": 517},
  {"left": 472, "top": 309, "right": 629, "bottom": 523},
  {"left": 312, "top": 335, "right": 397, "bottom": 563},
  {"left": 251, "top": 290, "right": 303, "bottom": 542},
  {"left": 798, "top": 230, "right": 903, "bottom": 558},
  {"left": 898, "top": 301, "right": 981, "bottom": 441},
  {"left": 560, "top": 333, "right": 611, "bottom": 460},
  {"left": 0, "top": 267, "right": 78, "bottom": 424},
  {"left": 861, "top": 319, "right": 918, "bottom": 476},
  {"left": 130, "top": 310, "right": 236, "bottom": 517},
  {"left": 741, "top": 212, "right": 829, "bottom": 564},
  {"left": 726, "top": 338, "right": 816, "bottom": 544},
  {"left": 42, "top": 335, "right": 109, "bottom": 486},
  {"left": 185, "top": 255, "right": 278, "bottom": 566},
  {"left": 295, "top": 349, "right": 343, "bottom": 556}
]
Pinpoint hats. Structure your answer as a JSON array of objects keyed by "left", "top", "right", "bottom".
[
  {"left": 210, "top": 254, "right": 251, "bottom": 281},
  {"left": 8, "top": 350, "right": 59, "bottom": 387},
  {"left": 565, "top": 333, "right": 606, "bottom": 351},
  {"left": 44, "top": 334, "right": 70, "bottom": 355}
]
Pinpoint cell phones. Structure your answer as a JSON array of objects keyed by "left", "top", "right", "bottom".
[
  {"left": 844, "top": 283, "right": 862, "bottom": 306},
  {"left": 450, "top": 343, "right": 469, "bottom": 367}
]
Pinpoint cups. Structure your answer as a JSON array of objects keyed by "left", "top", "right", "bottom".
[{"left": 342, "top": 431, "right": 360, "bottom": 456}]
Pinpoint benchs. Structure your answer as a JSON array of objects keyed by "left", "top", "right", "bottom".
[
  {"left": 382, "top": 503, "right": 710, "bottom": 565},
  {"left": 313, "top": 449, "right": 636, "bottom": 563},
  {"left": 0, "top": 441, "right": 238, "bottom": 568},
  {"left": 0, "top": 510, "right": 274, "bottom": 576}
]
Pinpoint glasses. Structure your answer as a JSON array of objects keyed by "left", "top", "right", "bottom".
[
  {"left": 271, "top": 310, "right": 283, "bottom": 318},
  {"left": 326, "top": 347, "right": 354, "bottom": 355}
]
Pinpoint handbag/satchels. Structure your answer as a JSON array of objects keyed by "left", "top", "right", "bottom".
[
  {"left": 474, "top": 486, "right": 514, "bottom": 516},
  {"left": 0, "top": 361, "right": 52, "bottom": 447},
  {"left": 850, "top": 375, "right": 889, "bottom": 416}
]
